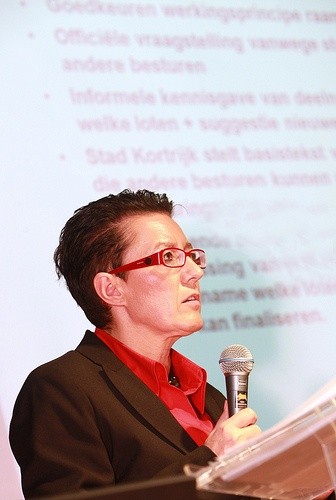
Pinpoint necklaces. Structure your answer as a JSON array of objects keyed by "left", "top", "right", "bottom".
[{"left": 166, "top": 374, "right": 177, "bottom": 384}]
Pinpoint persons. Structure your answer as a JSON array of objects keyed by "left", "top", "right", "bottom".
[{"left": 9, "top": 189, "right": 266, "bottom": 500}]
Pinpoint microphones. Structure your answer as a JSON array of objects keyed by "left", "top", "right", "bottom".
[{"left": 220, "top": 345, "right": 255, "bottom": 418}]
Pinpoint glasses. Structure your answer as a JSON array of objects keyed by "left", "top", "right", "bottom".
[{"left": 108, "top": 247, "right": 206, "bottom": 277}]
